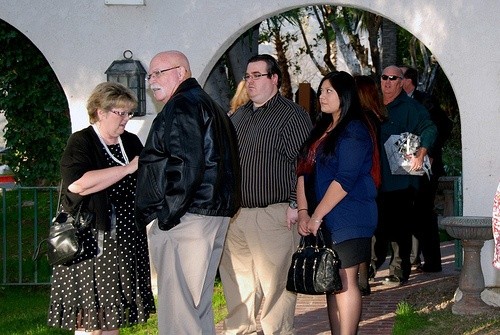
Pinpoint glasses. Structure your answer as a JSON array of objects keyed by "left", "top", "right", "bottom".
[
  {"left": 109, "top": 110, "right": 134, "bottom": 118},
  {"left": 243, "top": 72, "right": 271, "bottom": 80},
  {"left": 145, "top": 66, "right": 187, "bottom": 80},
  {"left": 380, "top": 74, "right": 402, "bottom": 80}
]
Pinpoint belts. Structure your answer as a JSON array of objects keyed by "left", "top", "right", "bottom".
[{"left": 242, "top": 204, "right": 268, "bottom": 209}]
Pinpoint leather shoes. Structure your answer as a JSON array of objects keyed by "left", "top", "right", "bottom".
[
  {"left": 358, "top": 265, "right": 375, "bottom": 280},
  {"left": 383, "top": 274, "right": 408, "bottom": 285}
]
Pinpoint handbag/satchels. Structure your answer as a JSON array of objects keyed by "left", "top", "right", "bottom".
[
  {"left": 286, "top": 229, "right": 343, "bottom": 295},
  {"left": 32, "top": 211, "right": 85, "bottom": 265}
]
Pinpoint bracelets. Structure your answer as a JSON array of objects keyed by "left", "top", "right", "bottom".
[
  {"left": 312, "top": 213, "right": 323, "bottom": 223},
  {"left": 297, "top": 208, "right": 308, "bottom": 212}
]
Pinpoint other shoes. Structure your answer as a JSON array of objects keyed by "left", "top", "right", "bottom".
[
  {"left": 416, "top": 264, "right": 424, "bottom": 271},
  {"left": 359, "top": 287, "right": 371, "bottom": 296}
]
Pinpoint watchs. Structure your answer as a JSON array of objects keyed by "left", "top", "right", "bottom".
[{"left": 289, "top": 201, "right": 298, "bottom": 210}]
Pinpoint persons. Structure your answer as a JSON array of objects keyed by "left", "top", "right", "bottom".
[
  {"left": 225, "top": 66, "right": 443, "bottom": 335},
  {"left": 143, "top": 51, "right": 242, "bottom": 335},
  {"left": 46, "top": 82, "right": 145, "bottom": 335},
  {"left": 217, "top": 54, "right": 313, "bottom": 335}
]
